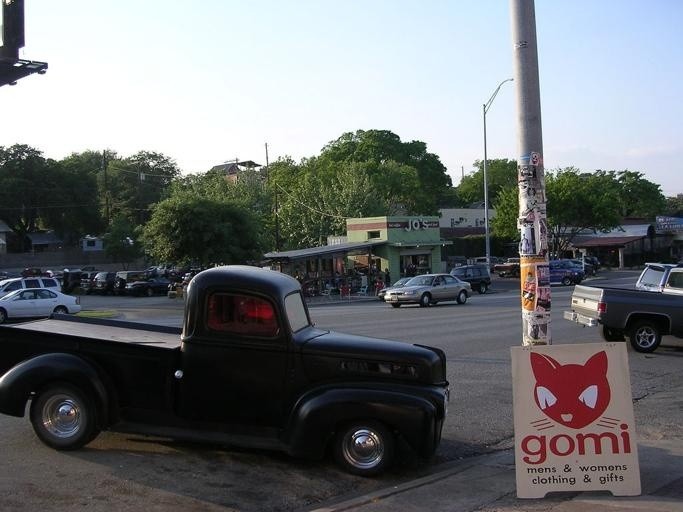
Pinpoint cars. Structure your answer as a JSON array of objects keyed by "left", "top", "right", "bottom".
[
  {"left": 0, "top": 275, "right": 81, "bottom": 325},
  {"left": 21, "top": 266, "right": 203, "bottom": 297},
  {"left": 377, "top": 273, "right": 473, "bottom": 309},
  {"left": 472, "top": 251, "right": 601, "bottom": 285}
]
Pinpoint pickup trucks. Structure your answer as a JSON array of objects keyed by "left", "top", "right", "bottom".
[{"left": 0, "top": 265, "right": 449, "bottom": 479}]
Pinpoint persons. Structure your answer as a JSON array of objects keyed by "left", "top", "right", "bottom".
[
  {"left": 526, "top": 187, "right": 542, "bottom": 212},
  {"left": 291, "top": 267, "right": 390, "bottom": 297},
  {"left": 521, "top": 233, "right": 530, "bottom": 254},
  {"left": 525, "top": 272, "right": 535, "bottom": 285},
  {"left": 433, "top": 276, "right": 445, "bottom": 286},
  {"left": 537, "top": 219, "right": 548, "bottom": 257}
]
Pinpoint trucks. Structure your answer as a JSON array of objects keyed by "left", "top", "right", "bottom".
[{"left": 563, "top": 262, "right": 683, "bottom": 353}]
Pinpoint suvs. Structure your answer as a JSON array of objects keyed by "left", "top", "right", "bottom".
[{"left": 449, "top": 266, "right": 492, "bottom": 295}]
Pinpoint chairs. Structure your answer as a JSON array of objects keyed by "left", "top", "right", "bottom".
[
  {"left": 320, "top": 287, "right": 334, "bottom": 301},
  {"left": 356, "top": 285, "right": 368, "bottom": 297}
]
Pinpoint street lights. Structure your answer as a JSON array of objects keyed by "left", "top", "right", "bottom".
[{"left": 482, "top": 78, "right": 517, "bottom": 287}]
